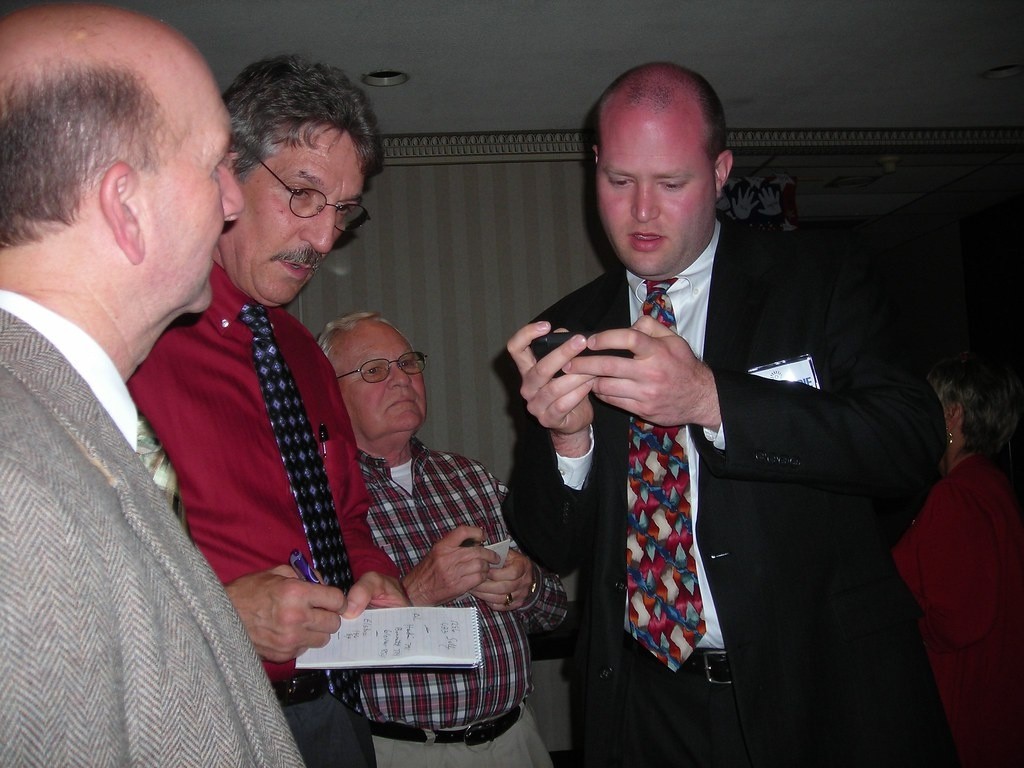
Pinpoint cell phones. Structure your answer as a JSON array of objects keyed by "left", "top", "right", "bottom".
[{"left": 532, "top": 332, "right": 634, "bottom": 379}]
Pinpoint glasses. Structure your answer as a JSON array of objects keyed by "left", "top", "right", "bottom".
[
  {"left": 248, "top": 146, "right": 372, "bottom": 232},
  {"left": 336, "top": 351, "right": 428, "bottom": 383}
]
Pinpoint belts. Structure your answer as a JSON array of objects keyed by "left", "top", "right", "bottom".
[
  {"left": 689, "top": 647, "right": 734, "bottom": 684},
  {"left": 272, "top": 670, "right": 329, "bottom": 707},
  {"left": 367, "top": 696, "right": 527, "bottom": 746}
]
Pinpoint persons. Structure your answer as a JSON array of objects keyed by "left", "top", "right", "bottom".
[
  {"left": 317, "top": 312, "right": 568, "bottom": 767},
  {"left": 500, "top": 63, "right": 959, "bottom": 768},
  {"left": 0, "top": 0, "right": 307, "bottom": 768},
  {"left": 892, "top": 354, "right": 1024, "bottom": 768},
  {"left": 125, "top": 56, "right": 409, "bottom": 768}
]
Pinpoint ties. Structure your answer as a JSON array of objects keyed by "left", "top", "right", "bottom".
[
  {"left": 238, "top": 299, "right": 356, "bottom": 597},
  {"left": 135, "top": 409, "right": 193, "bottom": 540},
  {"left": 624, "top": 274, "right": 708, "bottom": 675}
]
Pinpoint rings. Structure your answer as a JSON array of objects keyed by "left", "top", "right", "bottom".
[{"left": 505, "top": 593, "right": 513, "bottom": 607}]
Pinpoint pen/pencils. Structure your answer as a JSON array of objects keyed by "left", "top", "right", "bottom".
[
  {"left": 289, "top": 548, "right": 321, "bottom": 586},
  {"left": 463, "top": 536, "right": 478, "bottom": 548}
]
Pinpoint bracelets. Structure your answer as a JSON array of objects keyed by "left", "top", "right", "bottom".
[{"left": 525, "top": 568, "right": 536, "bottom": 601}]
